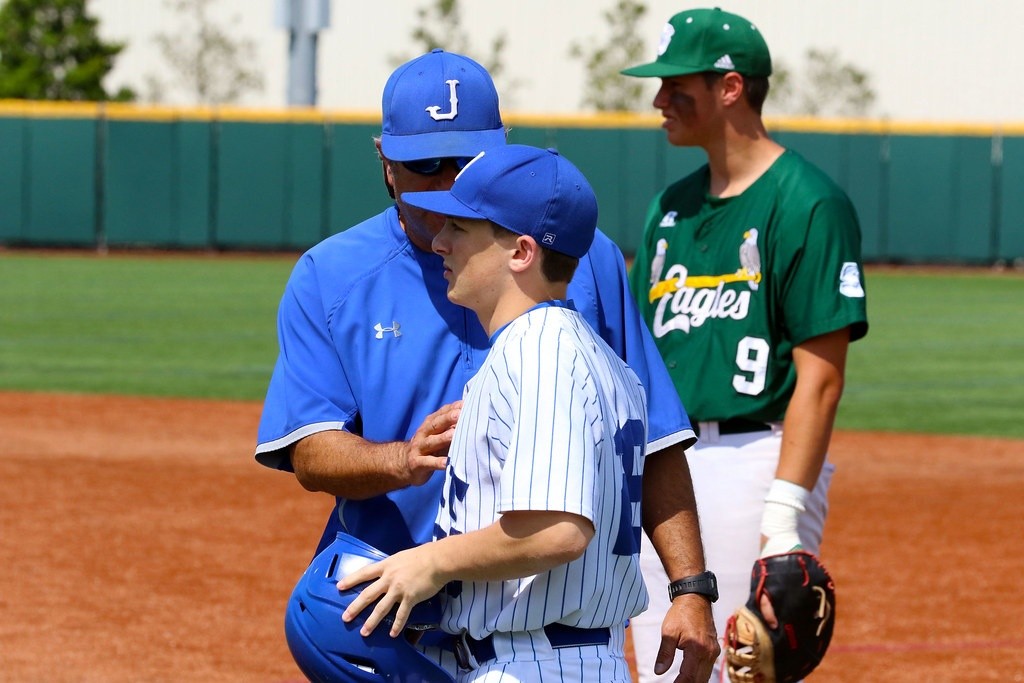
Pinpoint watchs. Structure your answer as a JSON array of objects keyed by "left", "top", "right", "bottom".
[{"left": 668, "top": 571, "right": 720, "bottom": 603}]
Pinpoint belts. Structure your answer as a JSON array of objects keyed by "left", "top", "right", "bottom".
[
  {"left": 451, "top": 623, "right": 610, "bottom": 671},
  {"left": 693, "top": 417, "right": 770, "bottom": 441}
]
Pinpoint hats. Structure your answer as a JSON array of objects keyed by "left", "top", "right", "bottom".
[
  {"left": 621, "top": 7, "right": 772, "bottom": 79},
  {"left": 400, "top": 144, "right": 598, "bottom": 259},
  {"left": 380, "top": 48, "right": 506, "bottom": 161}
]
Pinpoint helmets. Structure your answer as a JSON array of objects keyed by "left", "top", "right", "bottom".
[{"left": 284, "top": 529, "right": 455, "bottom": 683}]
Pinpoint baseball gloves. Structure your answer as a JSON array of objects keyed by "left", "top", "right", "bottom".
[{"left": 717, "top": 550, "right": 837, "bottom": 683}]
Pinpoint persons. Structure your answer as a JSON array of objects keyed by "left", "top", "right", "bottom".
[
  {"left": 619, "top": 6, "right": 868, "bottom": 683},
  {"left": 255, "top": 49, "right": 720, "bottom": 683},
  {"left": 338, "top": 144, "right": 651, "bottom": 683}
]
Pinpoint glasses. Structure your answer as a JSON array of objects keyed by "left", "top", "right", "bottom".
[{"left": 402, "top": 157, "right": 474, "bottom": 175}]
